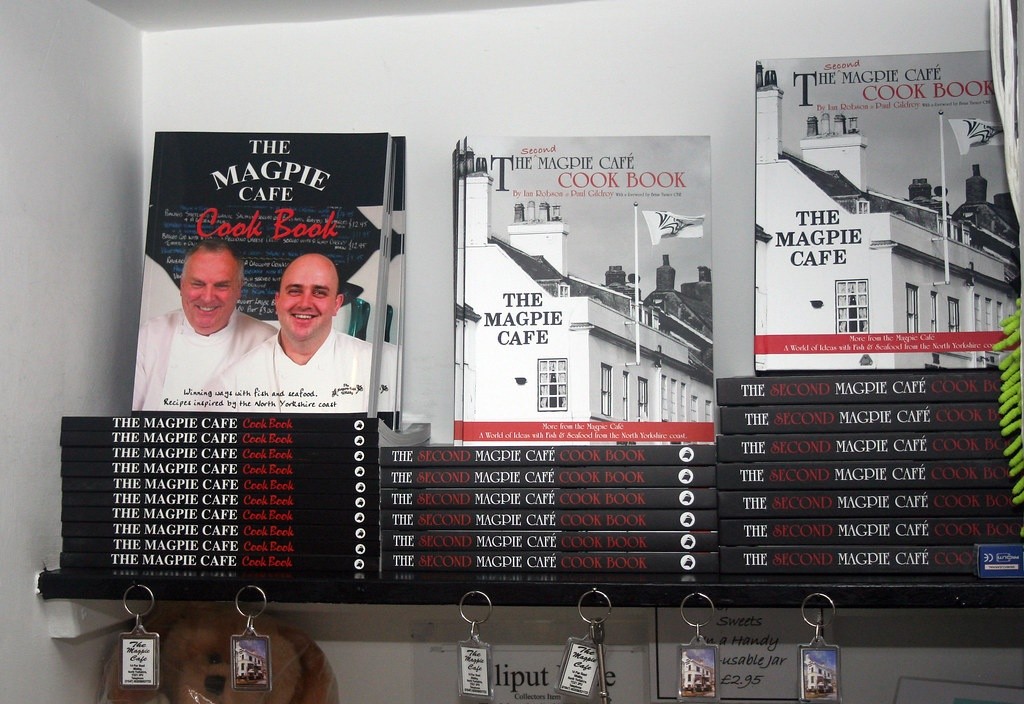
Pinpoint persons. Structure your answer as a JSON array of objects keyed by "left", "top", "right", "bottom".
[
  {"left": 133, "top": 239, "right": 278, "bottom": 411},
  {"left": 220, "top": 254, "right": 396, "bottom": 412}
]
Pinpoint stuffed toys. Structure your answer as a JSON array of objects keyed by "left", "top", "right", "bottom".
[{"left": 100, "top": 603, "right": 339, "bottom": 704}]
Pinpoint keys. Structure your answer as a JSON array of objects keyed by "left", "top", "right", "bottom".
[{"left": 590, "top": 617, "right": 612, "bottom": 704}]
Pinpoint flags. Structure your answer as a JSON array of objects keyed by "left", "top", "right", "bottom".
[
  {"left": 642, "top": 211, "right": 705, "bottom": 244},
  {"left": 949, "top": 119, "right": 1006, "bottom": 154}
]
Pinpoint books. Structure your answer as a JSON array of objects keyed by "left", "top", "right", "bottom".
[
  {"left": 452, "top": 134, "right": 715, "bottom": 442},
  {"left": 59, "top": 371, "right": 1024, "bottom": 582},
  {"left": 132, "top": 131, "right": 407, "bottom": 429},
  {"left": 753, "top": 50, "right": 1022, "bottom": 373}
]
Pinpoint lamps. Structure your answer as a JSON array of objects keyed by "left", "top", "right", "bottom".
[
  {"left": 515, "top": 377, "right": 526, "bottom": 384},
  {"left": 809, "top": 301, "right": 824, "bottom": 308}
]
